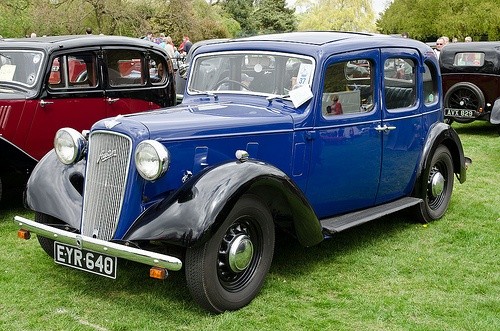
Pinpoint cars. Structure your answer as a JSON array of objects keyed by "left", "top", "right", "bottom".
[{"left": 435, "top": 40, "right": 500, "bottom": 126}]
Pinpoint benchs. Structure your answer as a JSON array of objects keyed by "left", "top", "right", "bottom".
[
  {"left": 343, "top": 83, "right": 414, "bottom": 112},
  {"left": 110, "top": 77, "right": 161, "bottom": 85}
]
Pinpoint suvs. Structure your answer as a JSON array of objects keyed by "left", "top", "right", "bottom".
[
  {"left": 13, "top": 31, "right": 471, "bottom": 313},
  {"left": 0, "top": 34, "right": 175, "bottom": 216}
]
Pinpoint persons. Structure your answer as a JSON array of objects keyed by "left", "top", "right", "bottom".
[
  {"left": 434, "top": 37, "right": 449, "bottom": 60},
  {"left": 86, "top": 27, "right": 92, "bottom": 35},
  {"left": 395, "top": 33, "right": 412, "bottom": 81},
  {"left": 25, "top": 33, "right": 37, "bottom": 38},
  {"left": 140, "top": 32, "right": 192, "bottom": 80},
  {"left": 454, "top": 38, "right": 457, "bottom": 43},
  {"left": 324, "top": 94, "right": 344, "bottom": 116},
  {"left": 462, "top": 37, "right": 476, "bottom": 65}
]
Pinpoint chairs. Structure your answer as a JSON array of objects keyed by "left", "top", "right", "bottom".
[{"left": 248, "top": 74, "right": 290, "bottom": 93}]
